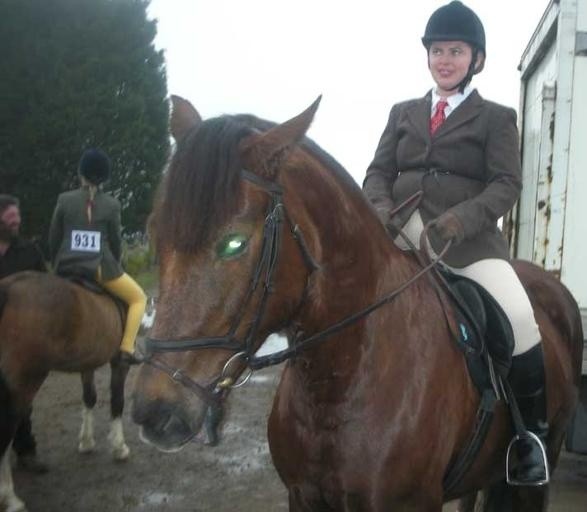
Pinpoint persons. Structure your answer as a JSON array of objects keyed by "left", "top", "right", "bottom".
[
  {"left": 48, "top": 150, "right": 147, "bottom": 363},
  {"left": 0, "top": 193, "right": 51, "bottom": 473},
  {"left": 361, "top": 1, "right": 551, "bottom": 482}
]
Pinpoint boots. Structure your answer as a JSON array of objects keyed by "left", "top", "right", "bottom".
[{"left": 504, "top": 338, "right": 552, "bottom": 486}]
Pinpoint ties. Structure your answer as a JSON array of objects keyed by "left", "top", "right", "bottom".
[{"left": 430, "top": 100, "right": 450, "bottom": 137}]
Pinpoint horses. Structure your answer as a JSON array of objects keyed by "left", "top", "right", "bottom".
[
  {"left": 129, "top": 92, "right": 585, "bottom": 512},
  {"left": 0, "top": 269, "right": 131, "bottom": 511}
]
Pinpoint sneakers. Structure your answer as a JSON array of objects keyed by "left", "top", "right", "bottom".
[{"left": 116, "top": 348, "right": 146, "bottom": 365}]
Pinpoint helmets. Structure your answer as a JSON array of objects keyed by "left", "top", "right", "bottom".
[
  {"left": 79, "top": 147, "right": 113, "bottom": 185},
  {"left": 420, "top": 0, "right": 485, "bottom": 51}
]
